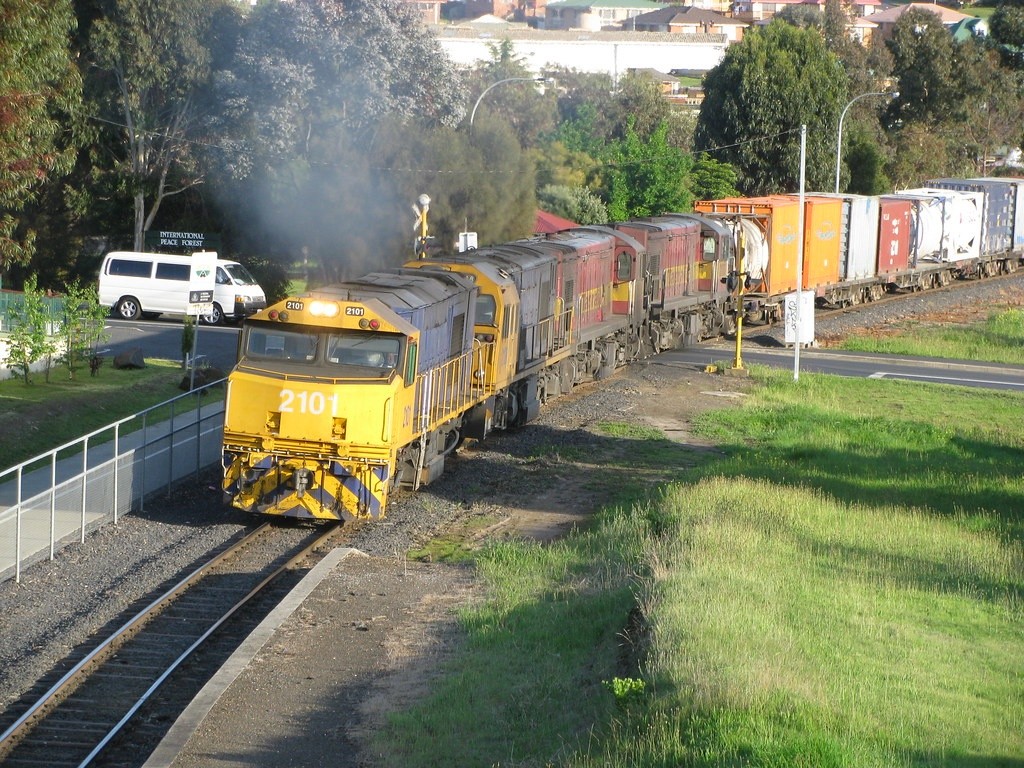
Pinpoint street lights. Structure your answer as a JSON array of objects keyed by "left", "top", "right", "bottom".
[
  {"left": 836, "top": 92, "right": 900, "bottom": 194},
  {"left": 464, "top": 78, "right": 554, "bottom": 232}
]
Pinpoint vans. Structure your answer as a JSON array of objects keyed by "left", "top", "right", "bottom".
[{"left": 96, "top": 251, "right": 267, "bottom": 327}]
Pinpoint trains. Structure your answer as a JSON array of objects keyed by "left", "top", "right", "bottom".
[{"left": 220, "top": 178, "right": 1024, "bottom": 524}]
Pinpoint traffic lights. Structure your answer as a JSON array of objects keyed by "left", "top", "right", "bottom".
[
  {"left": 414, "top": 238, "right": 442, "bottom": 256},
  {"left": 720, "top": 271, "right": 738, "bottom": 293}
]
